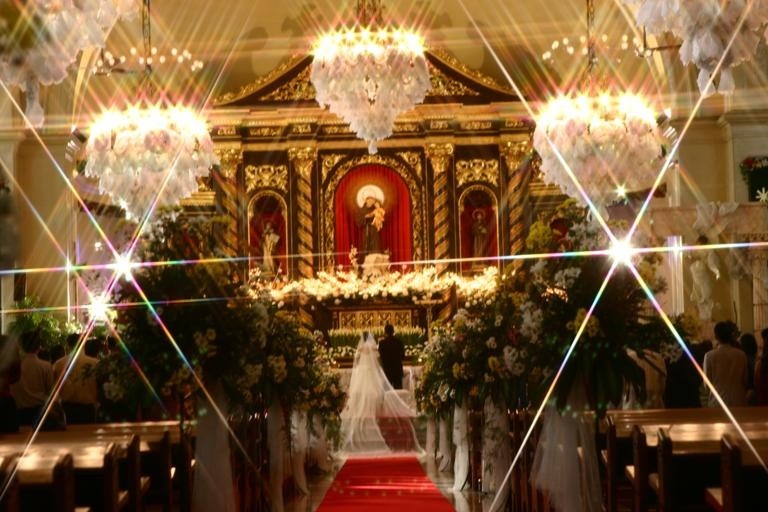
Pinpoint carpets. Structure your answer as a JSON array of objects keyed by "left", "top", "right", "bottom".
[{"left": 318, "top": 456, "right": 454, "bottom": 512}]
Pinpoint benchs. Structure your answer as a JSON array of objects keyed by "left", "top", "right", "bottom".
[
  {"left": 508, "top": 405, "right": 768, "bottom": 511},
  {"left": 0, "top": 418, "right": 265, "bottom": 512}
]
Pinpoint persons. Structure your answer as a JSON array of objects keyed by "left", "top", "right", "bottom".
[
  {"left": 470, "top": 213, "right": 489, "bottom": 265},
  {"left": 356, "top": 196, "right": 383, "bottom": 272},
  {"left": 620, "top": 319, "right": 767, "bottom": 410},
  {"left": 0, "top": 328, "right": 121, "bottom": 432},
  {"left": 370, "top": 199, "right": 386, "bottom": 232},
  {"left": 257, "top": 221, "right": 280, "bottom": 272},
  {"left": 353, "top": 330, "right": 380, "bottom": 401},
  {"left": 378, "top": 323, "right": 405, "bottom": 390}
]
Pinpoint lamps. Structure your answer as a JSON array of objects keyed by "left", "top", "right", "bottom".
[
  {"left": 533, "top": 1, "right": 677, "bottom": 226},
  {"left": 636, "top": 0, "right": 768, "bottom": 99},
  {"left": 83, "top": 0, "right": 216, "bottom": 234},
  {"left": 307, "top": 0, "right": 431, "bottom": 155}
]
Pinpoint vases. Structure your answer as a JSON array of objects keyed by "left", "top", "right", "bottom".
[
  {"left": 185, "top": 380, "right": 332, "bottom": 511},
  {"left": 748, "top": 169, "right": 768, "bottom": 202},
  {"left": 424, "top": 398, "right": 587, "bottom": 511}
]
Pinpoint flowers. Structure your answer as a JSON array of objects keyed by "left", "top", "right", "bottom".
[
  {"left": 739, "top": 154, "right": 768, "bottom": 183},
  {"left": 94, "top": 209, "right": 347, "bottom": 443},
  {"left": 400, "top": 200, "right": 680, "bottom": 418}
]
